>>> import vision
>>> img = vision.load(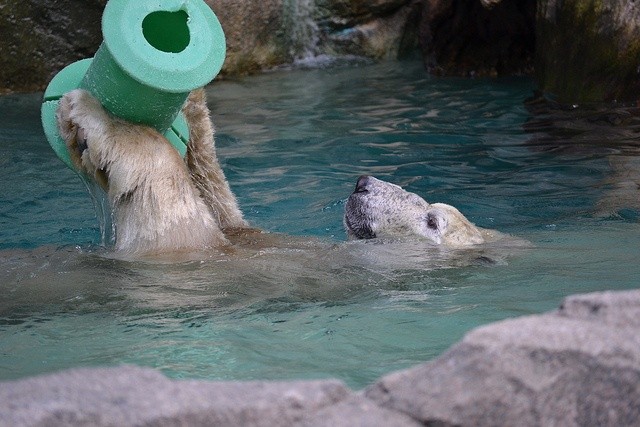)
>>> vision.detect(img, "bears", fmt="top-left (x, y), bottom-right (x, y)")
top-left (0, 86), bottom-right (525, 313)
